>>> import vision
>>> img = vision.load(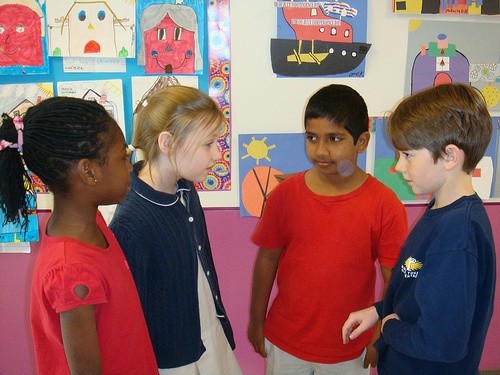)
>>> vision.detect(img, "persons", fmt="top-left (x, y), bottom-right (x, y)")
top-left (0, 95), bottom-right (160, 375)
top-left (247, 84), bottom-right (408, 375)
top-left (108, 85), bottom-right (246, 375)
top-left (341, 82), bottom-right (496, 375)
